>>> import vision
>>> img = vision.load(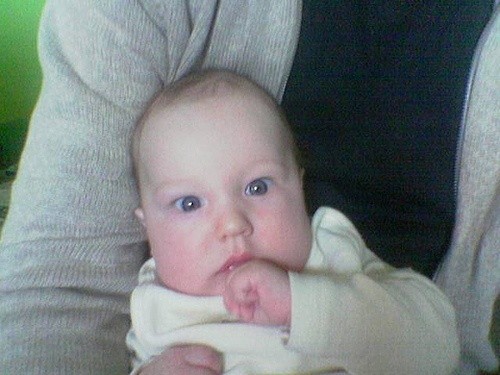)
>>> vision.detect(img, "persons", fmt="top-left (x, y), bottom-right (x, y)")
top-left (124, 66), bottom-right (459, 375)
top-left (0, 0), bottom-right (498, 374)
